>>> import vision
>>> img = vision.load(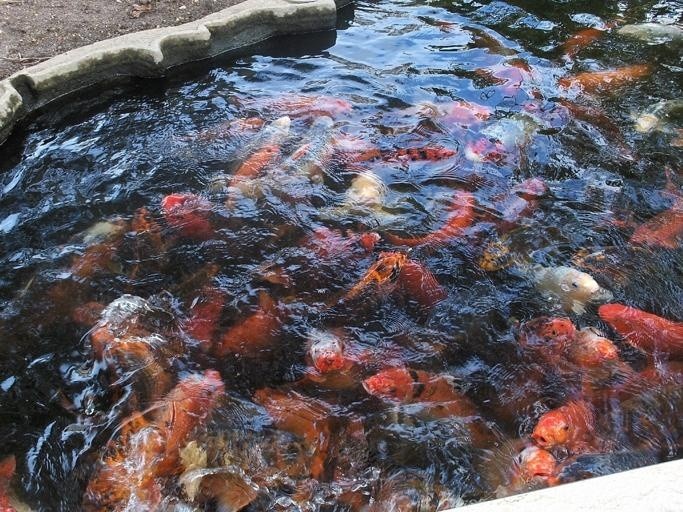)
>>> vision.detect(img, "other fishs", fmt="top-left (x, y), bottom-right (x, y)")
top-left (0, 16), bottom-right (683, 512)
